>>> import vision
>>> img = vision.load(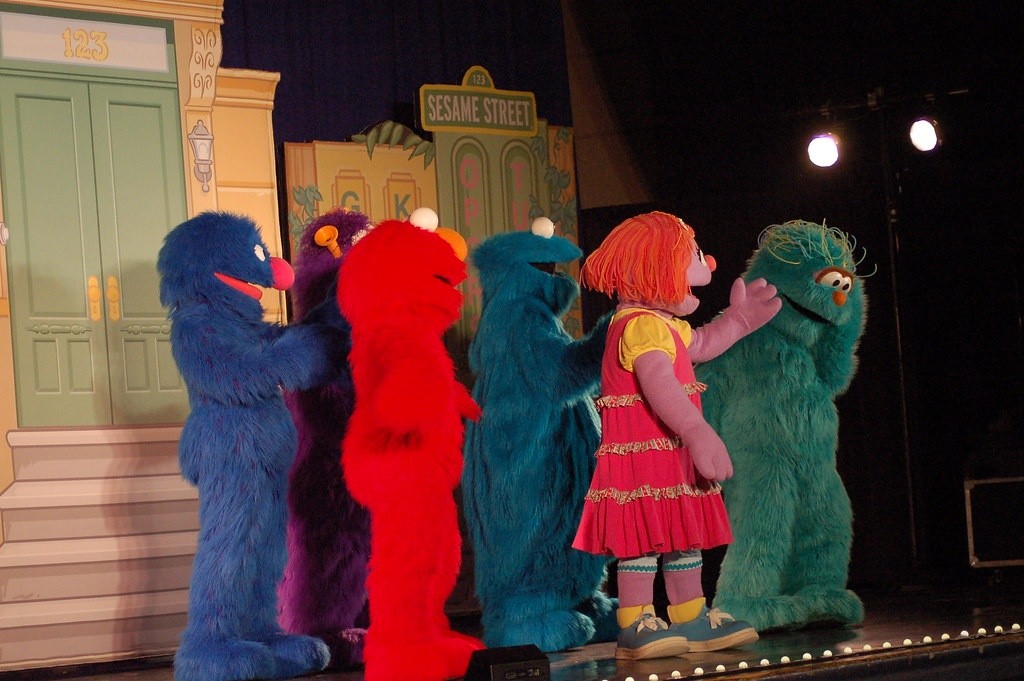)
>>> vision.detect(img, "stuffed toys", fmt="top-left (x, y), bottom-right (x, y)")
top-left (461, 217), bottom-right (626, 653)
top-left (334, 205), bottom-right (483, 681)
top-left (568, 208), bottom-right (781, 661)
top-left (154, 210), bottom-right (351, 681)
top-left (276, 212), bottom-right (376, 673)
top-left (691, 216), bottom-right (880, 634)
